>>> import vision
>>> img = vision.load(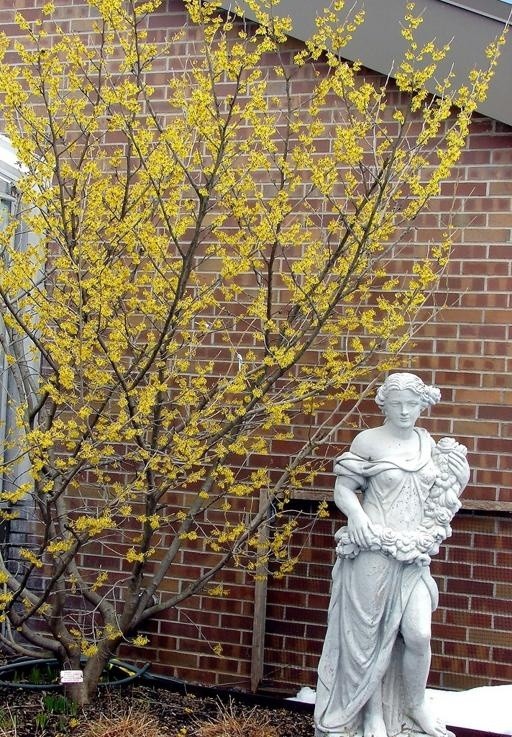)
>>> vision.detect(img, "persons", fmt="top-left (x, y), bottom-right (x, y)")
top-left (314, 372), bottom-right (472, 737)
